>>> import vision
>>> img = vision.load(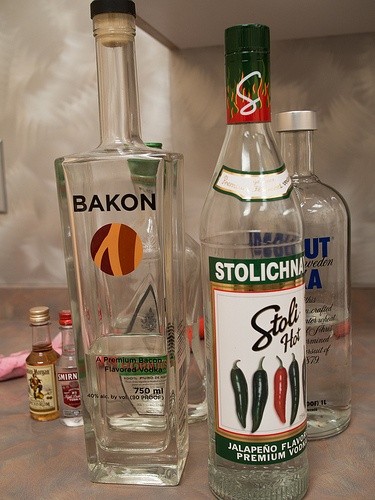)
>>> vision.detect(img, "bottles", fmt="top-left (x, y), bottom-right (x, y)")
top-left (144, 143), bottom-right (210, 427)
top-left (54, 311), bottom-right (85, 427)
top-left (54, 2), bottom-right (188, 486)
top-left (276, 111), bottom-right (353, 440)
top-left (198, 23), bottom-right (309, 499)
top-left (26, 307), bottom-right (61, 421)
